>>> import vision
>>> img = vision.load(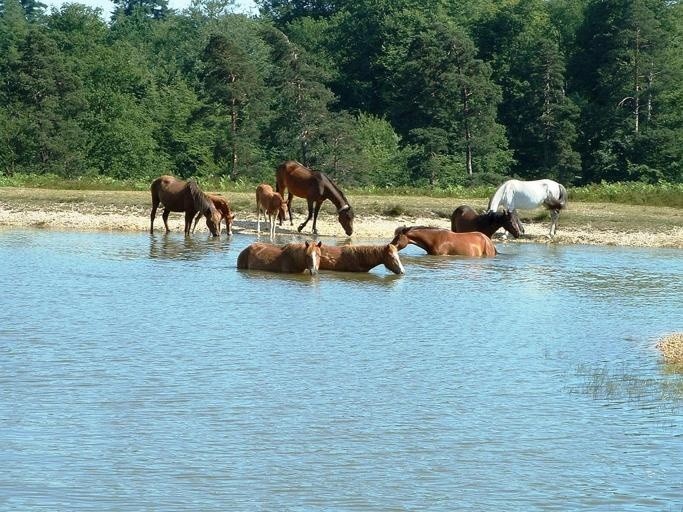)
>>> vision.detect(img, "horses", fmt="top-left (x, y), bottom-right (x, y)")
top-left (487, 177), bottom-right (568, 243)
top-left (187, 193), bottom-right (236, 236)
top-left (318, 242), bottom-right (405, 276)
top-left (390, 224), bottom-right (497, 258)
top-left (255, 183), bottom-right (290, 243)
top-left (149, 175), bottom-right (219, 240)
top-left (275, 160), bottom-right (356, 237)
top-left (454, 205), bottom-right (521, 241)
top-left (237, 240), bottom-right (323, 274)
